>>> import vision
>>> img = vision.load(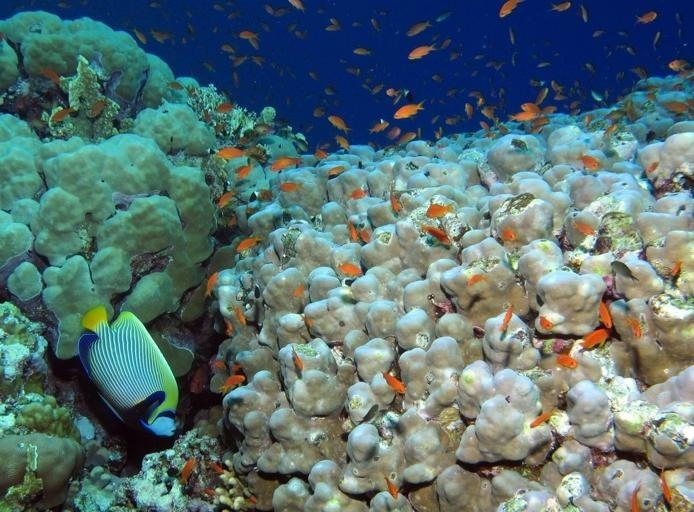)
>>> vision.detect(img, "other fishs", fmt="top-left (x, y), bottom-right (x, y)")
top-left (181, 0), bottom-right (694, 398)
